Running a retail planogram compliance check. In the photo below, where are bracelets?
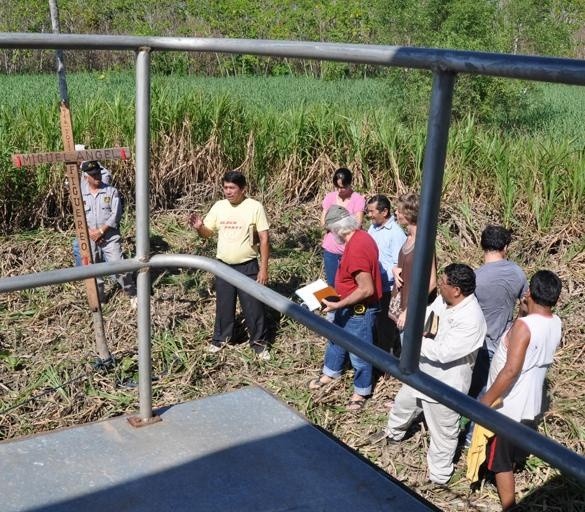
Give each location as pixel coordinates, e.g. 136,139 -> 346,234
261,266 -> 267,270
99,229 -> 105,236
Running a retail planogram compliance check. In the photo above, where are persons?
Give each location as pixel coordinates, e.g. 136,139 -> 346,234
188,171 -> 271,362
478,270 -> 563,512
367,195 -> 406,358
319,168 -> 366,321
66,144 -> 112,267
368,263 -> 488,493
463,226 -> 529,452
391,195 -> 438,349
79,160 -> 137,310
306,206 -> 382,413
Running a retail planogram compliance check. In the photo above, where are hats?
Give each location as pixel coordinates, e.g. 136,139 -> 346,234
323,205 -> 350,225
81,161 -> 103,172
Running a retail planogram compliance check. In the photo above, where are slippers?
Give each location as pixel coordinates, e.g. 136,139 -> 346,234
345,398 -> 367,410
309,379 -> 326,391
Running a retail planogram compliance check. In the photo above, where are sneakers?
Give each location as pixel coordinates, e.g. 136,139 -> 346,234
209,336 -> 231,353
369,431 -> 400,444
130,297 -> 155,316
258,351 -> 271,361
